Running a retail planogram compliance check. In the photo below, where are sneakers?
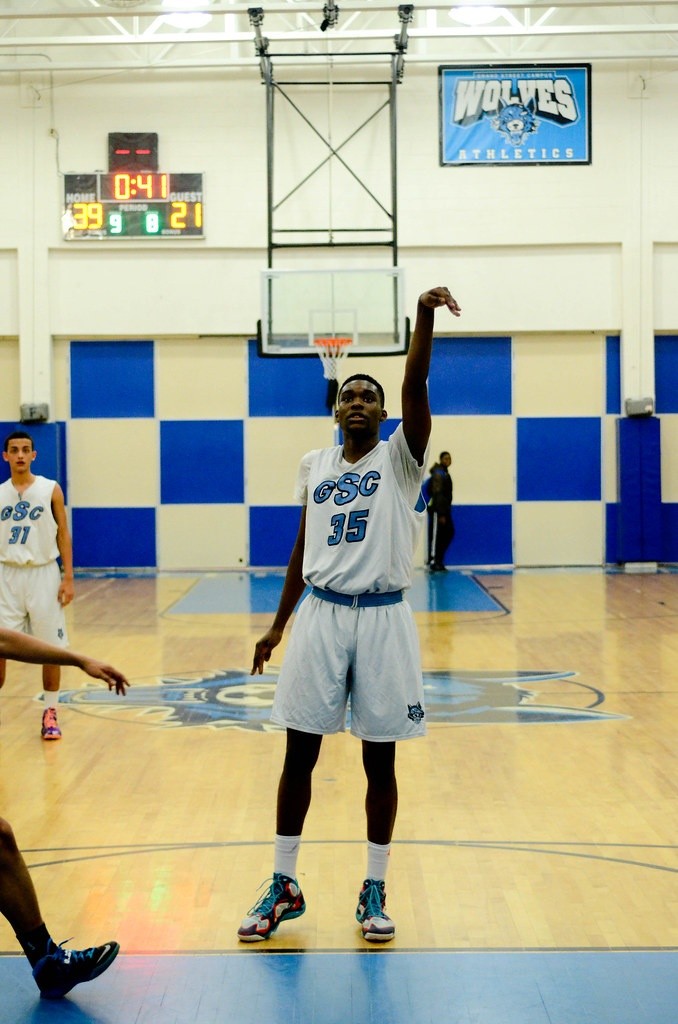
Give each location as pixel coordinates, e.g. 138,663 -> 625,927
41,707 -> 63,739
33,940 -> 119,998
356,877 -> 395,943
239,872 -> 304,942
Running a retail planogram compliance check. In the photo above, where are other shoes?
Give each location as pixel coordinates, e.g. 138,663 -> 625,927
430,564 -> 447,572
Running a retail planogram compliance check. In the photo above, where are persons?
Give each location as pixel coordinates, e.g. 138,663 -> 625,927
237,286 -> 461,944
0,431 -> 76,740
427,452 -> 455,573
0,625 -> 132,996
